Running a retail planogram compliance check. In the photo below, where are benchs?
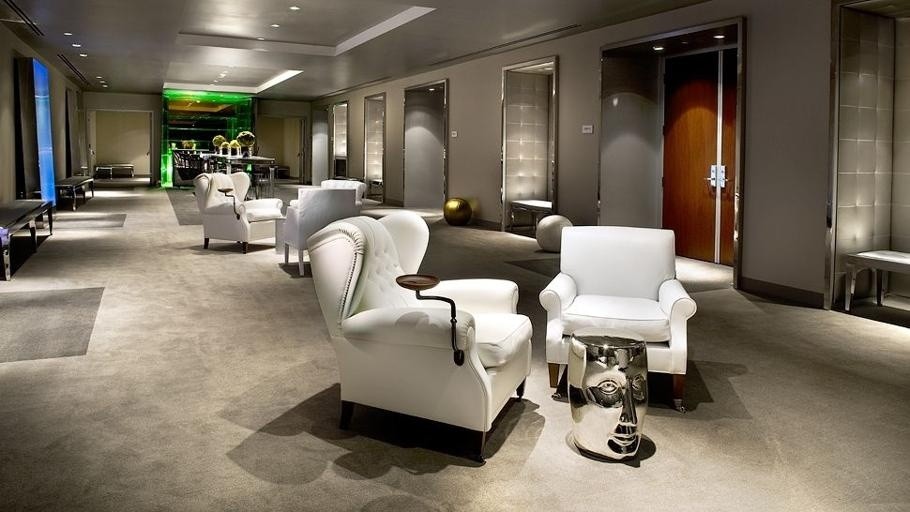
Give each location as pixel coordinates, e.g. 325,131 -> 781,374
844,250 -> 910,313
0,176 -> 95,281
94,163 -> 135,179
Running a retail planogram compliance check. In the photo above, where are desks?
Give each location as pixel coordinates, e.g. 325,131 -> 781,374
200,153 -> 276,174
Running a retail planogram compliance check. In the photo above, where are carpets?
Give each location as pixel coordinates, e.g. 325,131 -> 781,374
166,186 -> 204,226
0,286 -> 106,363
51,213 -> 127,228
